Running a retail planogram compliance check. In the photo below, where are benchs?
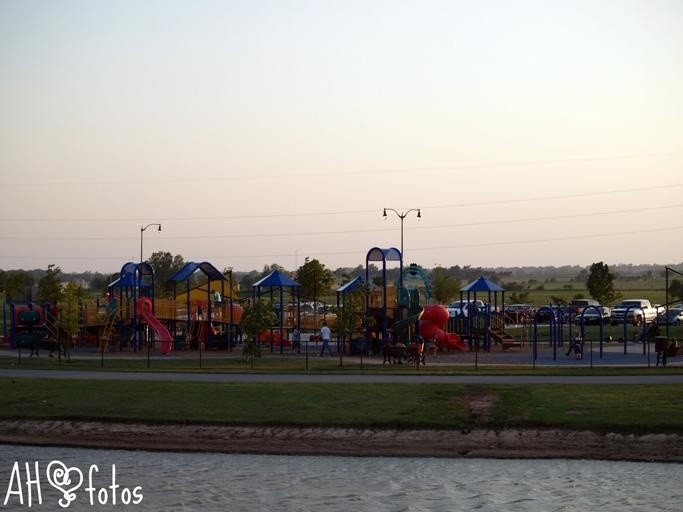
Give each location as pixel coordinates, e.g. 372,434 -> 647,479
662,346 -> 683,364
27,341 -> 61,358
383,347 -> 425,368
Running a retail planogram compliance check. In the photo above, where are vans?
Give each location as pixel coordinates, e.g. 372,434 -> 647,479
445,300 -> 485,319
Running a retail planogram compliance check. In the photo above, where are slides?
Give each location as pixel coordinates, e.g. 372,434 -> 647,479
142,314 -> 172,354
420,305 -> 467,353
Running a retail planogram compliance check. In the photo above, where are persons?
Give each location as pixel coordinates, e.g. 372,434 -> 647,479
29,332 -> 70,359
289,326 -> 302,352
314,320 -> 336,358
210,290 -> 222,309
381,332 -> 439,367
473,302 -> 583,362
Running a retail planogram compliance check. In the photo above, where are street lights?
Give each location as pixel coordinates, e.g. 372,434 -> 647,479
138,224 -> 162,352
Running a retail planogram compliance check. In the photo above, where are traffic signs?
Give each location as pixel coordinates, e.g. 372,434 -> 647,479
381,208 -> 421,364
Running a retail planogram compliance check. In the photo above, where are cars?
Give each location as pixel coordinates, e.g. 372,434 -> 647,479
171,302 -> 345,333
483,298 -> 683,326
